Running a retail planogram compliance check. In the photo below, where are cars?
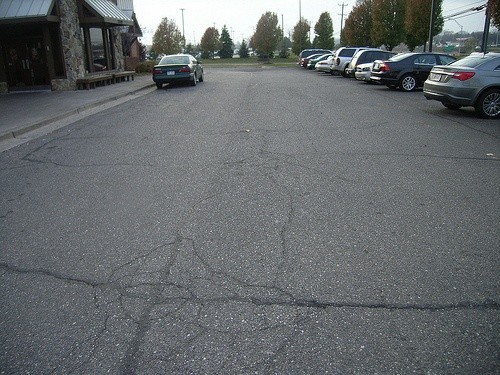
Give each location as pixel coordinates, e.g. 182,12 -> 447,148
153,55 -> 204,88
301,53 -> 334,73
345,50 -> 411,84
420,53 -> 500,119
370,52 -> 462,92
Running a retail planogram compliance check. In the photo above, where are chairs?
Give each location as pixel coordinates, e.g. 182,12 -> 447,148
421,56 -> 429,63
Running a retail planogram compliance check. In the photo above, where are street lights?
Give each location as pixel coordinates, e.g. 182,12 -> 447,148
181,9 -> 185,36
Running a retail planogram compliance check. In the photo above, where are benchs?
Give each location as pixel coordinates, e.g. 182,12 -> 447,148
106,71 -> 136,84
77,74 -> 113,90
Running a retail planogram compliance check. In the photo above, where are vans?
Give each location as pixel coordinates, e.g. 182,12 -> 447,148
299,49 -> 334,67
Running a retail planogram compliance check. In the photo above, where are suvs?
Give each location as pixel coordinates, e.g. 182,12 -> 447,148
330,46 -> 379,77
